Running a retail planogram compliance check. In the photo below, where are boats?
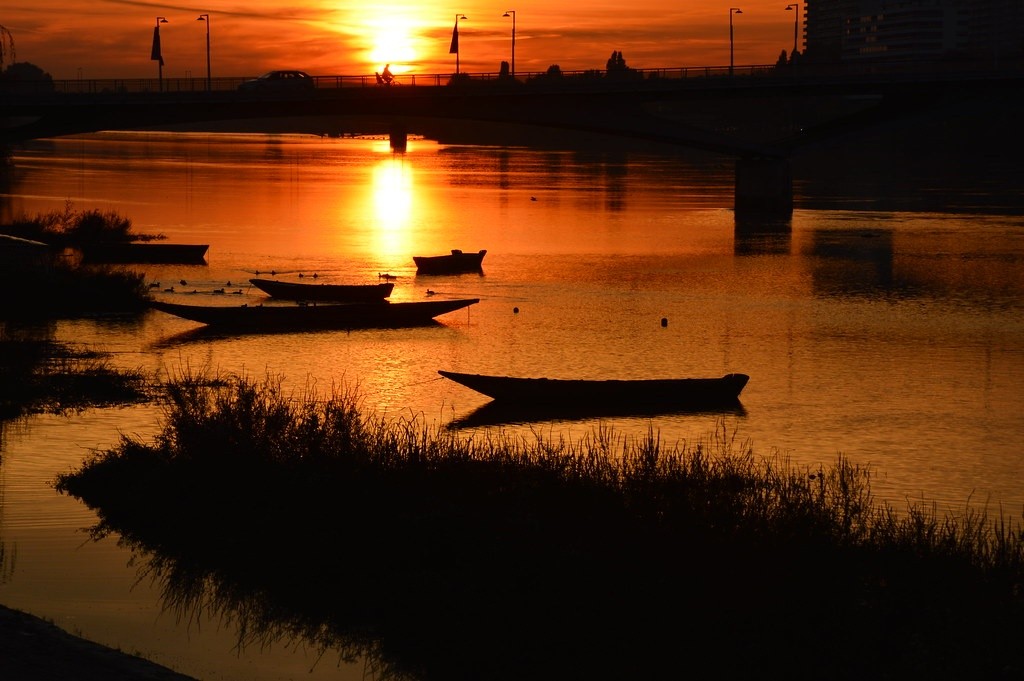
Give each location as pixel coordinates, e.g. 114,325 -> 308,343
437,369 -> 750,417
79,242 -> 210,262
246,279 -> 395,302
412,248 -> 488,277
147,297 -> 480,331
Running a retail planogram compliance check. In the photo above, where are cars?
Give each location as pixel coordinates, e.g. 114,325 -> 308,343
237,69 -> 315,93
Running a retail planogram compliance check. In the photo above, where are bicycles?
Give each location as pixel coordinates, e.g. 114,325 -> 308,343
375,77 -> 401,87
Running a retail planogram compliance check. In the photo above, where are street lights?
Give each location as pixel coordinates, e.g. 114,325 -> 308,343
455,13 -> 467,75
730,8 -> 744,69
197,14 -> 212,95
784,4 -> 799,57
157,15 -> 168,93
502,11 -> 516,75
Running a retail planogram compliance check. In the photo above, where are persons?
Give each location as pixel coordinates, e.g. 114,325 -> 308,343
375,72 -> 384,89
382,64 -> 394,86
